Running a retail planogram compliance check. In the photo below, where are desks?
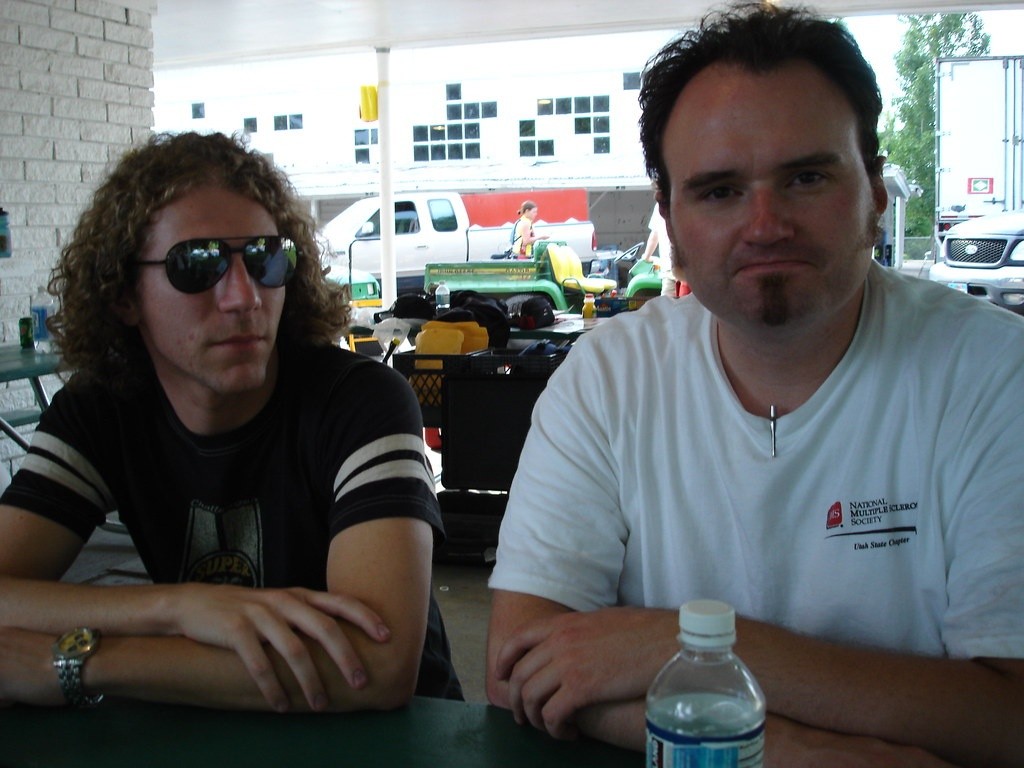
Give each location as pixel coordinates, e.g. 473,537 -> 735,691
0,691 -> 784,768
0,345 -> 74,415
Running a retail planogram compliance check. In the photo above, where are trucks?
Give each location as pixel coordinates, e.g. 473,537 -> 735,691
934,55 -> 1024,239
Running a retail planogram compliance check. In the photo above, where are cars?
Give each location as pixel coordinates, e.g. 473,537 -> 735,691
928,208 -> 1024,315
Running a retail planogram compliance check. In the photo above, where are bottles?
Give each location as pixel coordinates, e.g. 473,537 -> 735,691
582,294 -> 597,318
434,280 -> 450,309
645,599 -> 767,768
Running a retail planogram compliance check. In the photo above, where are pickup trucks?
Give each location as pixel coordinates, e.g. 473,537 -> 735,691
311,190 -> 598,293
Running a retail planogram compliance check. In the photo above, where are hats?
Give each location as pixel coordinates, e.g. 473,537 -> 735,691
374,293 -> 434,323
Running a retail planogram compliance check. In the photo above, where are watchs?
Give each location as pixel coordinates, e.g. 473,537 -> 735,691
52,626 -> 105,707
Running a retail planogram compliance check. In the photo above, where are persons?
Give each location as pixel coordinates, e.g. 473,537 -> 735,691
486,9 -> 1024,768
512,201 -> 550,259
641,201 -> 678,297
0,132 -> 466,714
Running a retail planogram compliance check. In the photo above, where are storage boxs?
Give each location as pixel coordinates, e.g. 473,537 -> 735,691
391,349 -> 573,426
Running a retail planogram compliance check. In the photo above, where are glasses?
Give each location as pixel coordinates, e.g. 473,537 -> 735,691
141,236 -> 299,294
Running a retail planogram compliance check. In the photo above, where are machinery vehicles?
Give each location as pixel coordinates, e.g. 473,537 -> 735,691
423,239 -> 662,316
318,263 -> 384,309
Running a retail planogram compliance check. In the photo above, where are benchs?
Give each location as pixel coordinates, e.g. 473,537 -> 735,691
0,409 -> 40,451
